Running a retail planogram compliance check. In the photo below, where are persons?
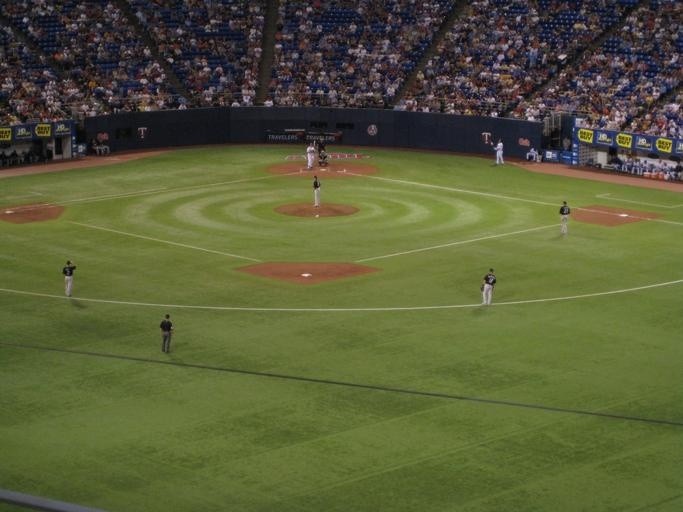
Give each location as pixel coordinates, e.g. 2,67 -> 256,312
559,200 -> 570,232
0,1 -> 683,183
159,314 -> 173,354
480,267 -> 496,306
312,175 -> 321,207
62,259 -> 76,297
